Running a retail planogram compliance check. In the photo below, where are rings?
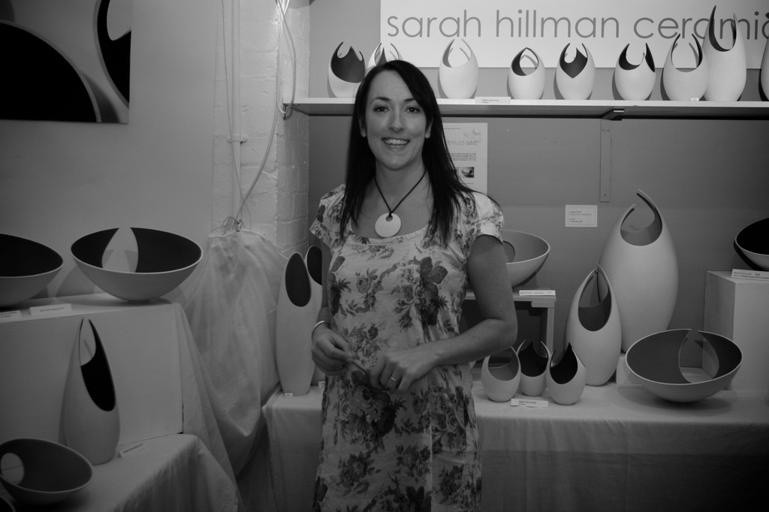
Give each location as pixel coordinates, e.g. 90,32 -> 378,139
391,376 -> 397,382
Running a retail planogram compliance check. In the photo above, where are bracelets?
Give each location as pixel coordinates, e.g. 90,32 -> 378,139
311,320 -> 330,345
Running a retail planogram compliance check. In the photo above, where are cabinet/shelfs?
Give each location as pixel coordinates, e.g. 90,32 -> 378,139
4,282 -> 198,511
250,96 -> 769,507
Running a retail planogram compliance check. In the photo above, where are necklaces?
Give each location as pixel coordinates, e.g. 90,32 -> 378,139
375,167 -> 428,238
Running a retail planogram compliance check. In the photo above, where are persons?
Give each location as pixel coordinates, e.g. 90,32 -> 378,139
311,59 -> 518,512
